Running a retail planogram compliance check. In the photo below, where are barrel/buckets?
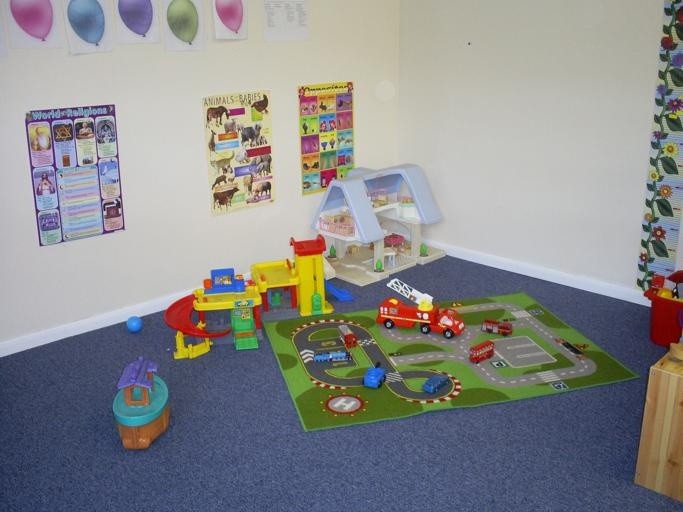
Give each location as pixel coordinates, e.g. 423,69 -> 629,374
645,288 -> 683,346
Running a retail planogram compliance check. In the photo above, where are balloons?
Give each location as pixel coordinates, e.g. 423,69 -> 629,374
10,0 -> 53,42
214,0 -> 243,35
165,1 -> 198,45
66,1 -> 104,48
117,0 -> 152,38
